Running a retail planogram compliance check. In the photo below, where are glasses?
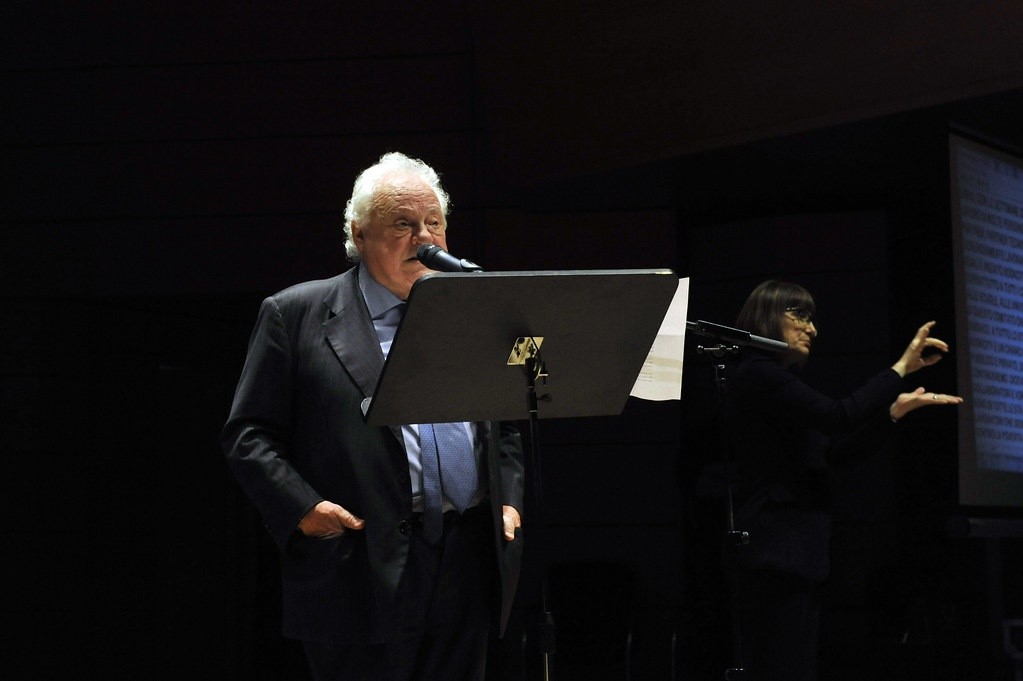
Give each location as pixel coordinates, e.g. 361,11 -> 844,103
786,305 -> 811,322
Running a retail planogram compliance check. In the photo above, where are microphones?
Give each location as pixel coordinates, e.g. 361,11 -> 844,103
417,244 -> 484,275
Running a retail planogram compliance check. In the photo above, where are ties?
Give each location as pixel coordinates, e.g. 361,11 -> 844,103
398,303 -> 480,546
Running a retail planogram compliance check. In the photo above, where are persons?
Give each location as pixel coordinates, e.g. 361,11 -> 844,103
700,279 -> 965,681
219,153 -> 527,681
